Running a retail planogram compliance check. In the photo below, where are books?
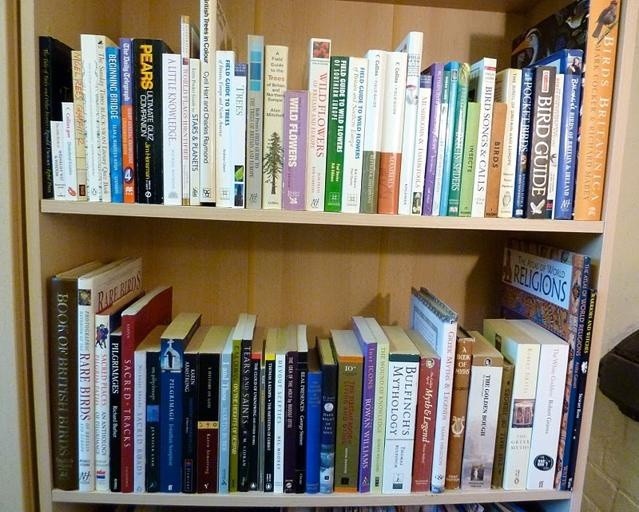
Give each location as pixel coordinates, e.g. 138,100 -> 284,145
44,242 -> 597,512
38,2 -> 621,221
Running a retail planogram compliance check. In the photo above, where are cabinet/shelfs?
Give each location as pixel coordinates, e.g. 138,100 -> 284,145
0,0 -> 639,512
